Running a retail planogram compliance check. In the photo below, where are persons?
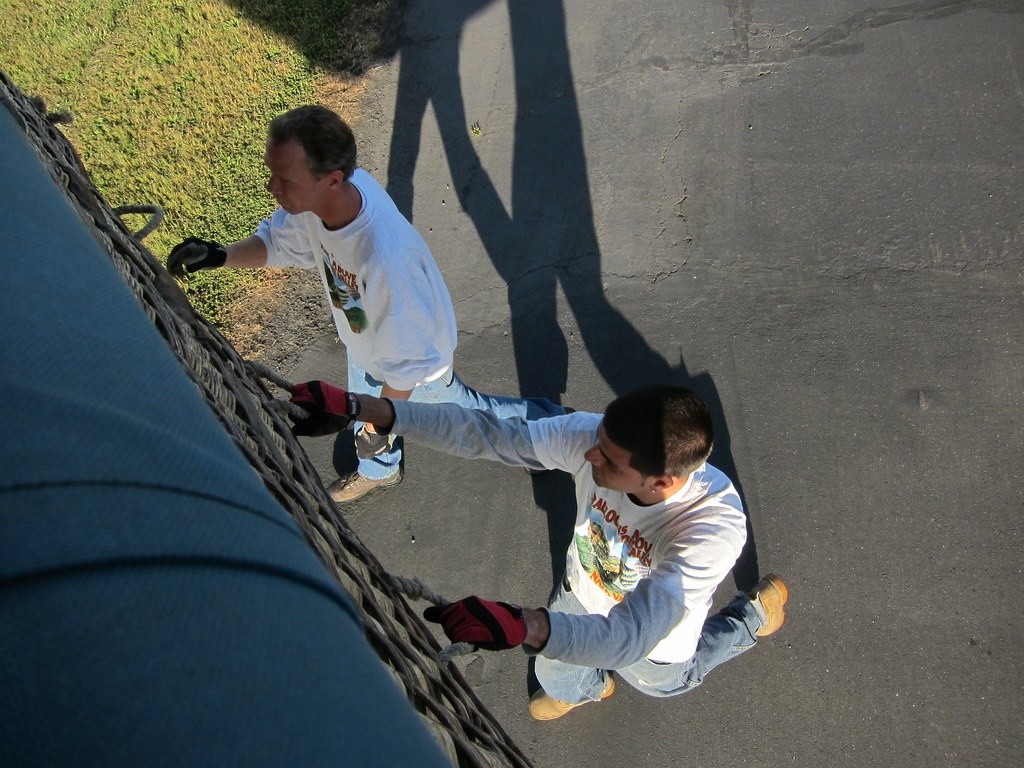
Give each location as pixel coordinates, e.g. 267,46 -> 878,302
288,384 -> 788,719
167,103 -> 457,504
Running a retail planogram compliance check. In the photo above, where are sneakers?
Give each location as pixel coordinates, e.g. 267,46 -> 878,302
325,467 -> 403,505
564,406 -> 576,414
527,670 -> 615,721
749,573 -> 788,636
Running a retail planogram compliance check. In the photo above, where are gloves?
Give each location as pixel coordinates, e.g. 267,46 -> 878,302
422,595 -> 528,653
286,380 -> 361,437
354,424 -> 393,459
166,235 -> 227,284
